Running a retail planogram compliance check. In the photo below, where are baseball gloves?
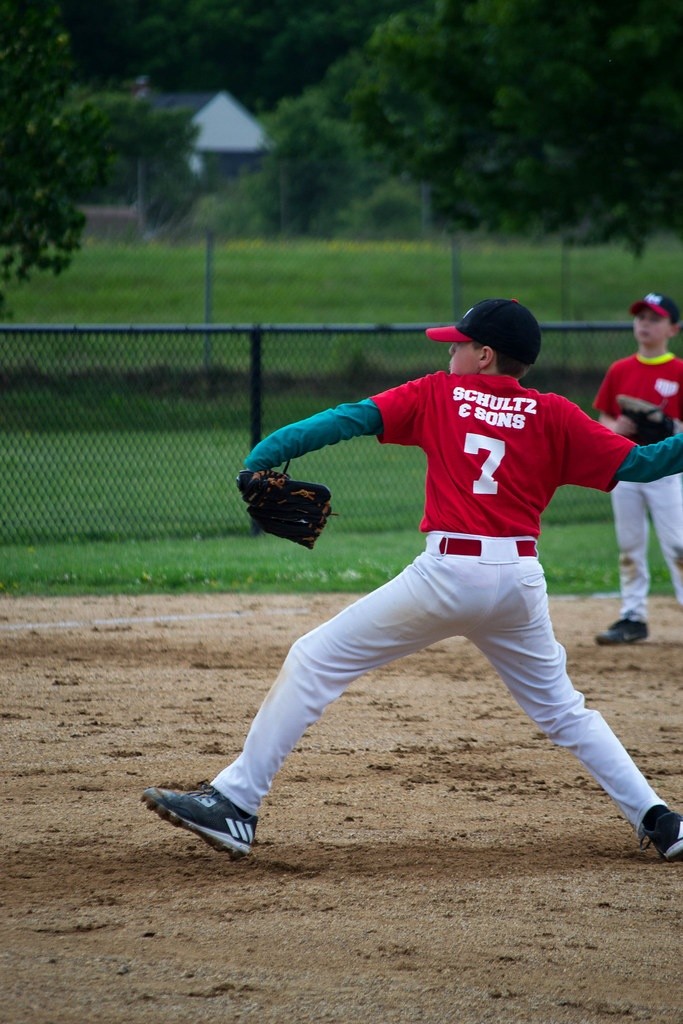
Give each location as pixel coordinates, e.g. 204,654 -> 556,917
236,458 -> 339,550
617,395 -> 674,444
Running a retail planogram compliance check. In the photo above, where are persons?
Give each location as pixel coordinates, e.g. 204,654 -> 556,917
592,293 -> 683,646
140,299 -> 683,858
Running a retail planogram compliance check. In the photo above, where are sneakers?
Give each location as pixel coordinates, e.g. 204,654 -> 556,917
141,784 -> 258,860
640,812 -> 683,860
596,617 -> 647,643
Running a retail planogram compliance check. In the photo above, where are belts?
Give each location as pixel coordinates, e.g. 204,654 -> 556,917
439,537 -> 536,557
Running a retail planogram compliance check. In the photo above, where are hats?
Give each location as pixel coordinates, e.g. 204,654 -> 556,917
425,298 -> 541,364
628,292 -> 679,324
